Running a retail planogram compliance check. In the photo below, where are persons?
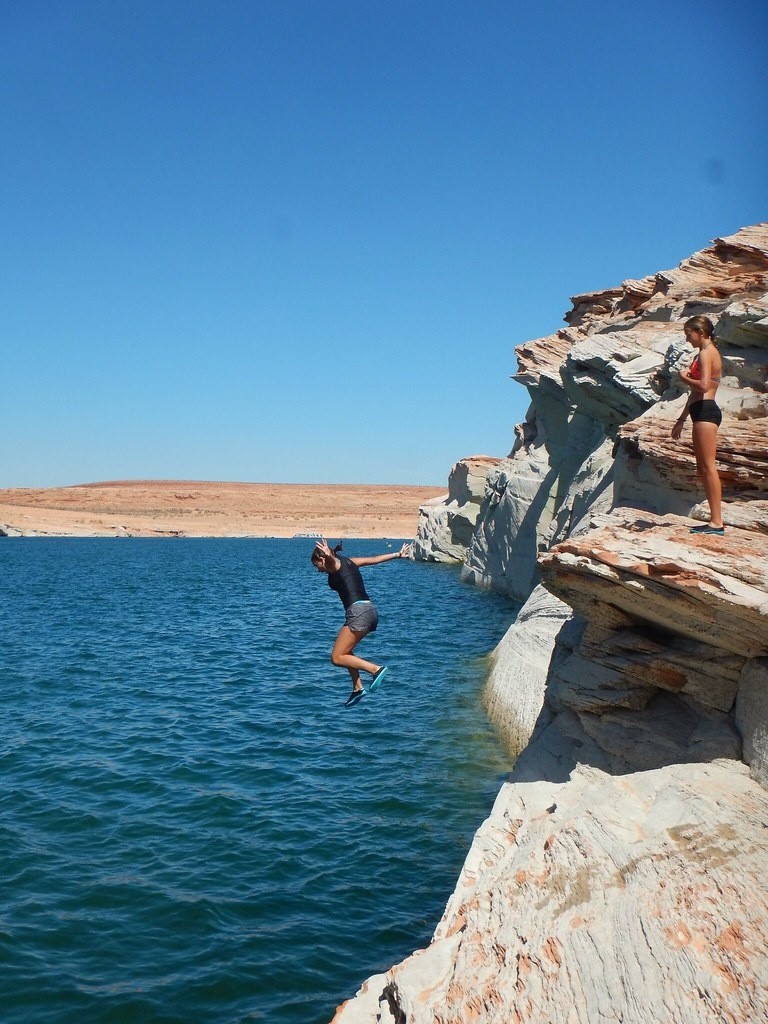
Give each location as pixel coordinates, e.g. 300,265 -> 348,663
311,539 -> 412,708
671,316 -> 732,537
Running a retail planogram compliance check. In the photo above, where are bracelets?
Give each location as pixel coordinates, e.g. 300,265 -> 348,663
676,418 -> 687,422
393,550 -> 402,559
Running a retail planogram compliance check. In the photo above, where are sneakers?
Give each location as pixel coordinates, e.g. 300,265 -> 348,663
344,687 -> 367,707
690,524 -> 724,536
369,666 -> 389,693
